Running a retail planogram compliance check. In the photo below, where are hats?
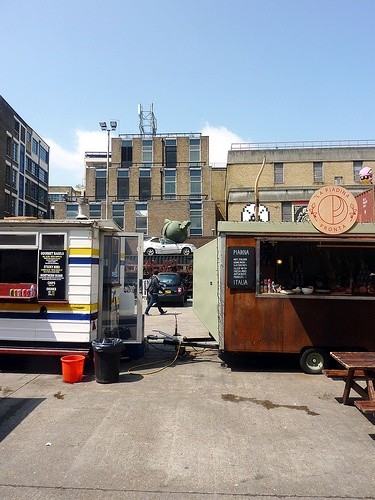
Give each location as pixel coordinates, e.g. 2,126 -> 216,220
152,269 -> 160,273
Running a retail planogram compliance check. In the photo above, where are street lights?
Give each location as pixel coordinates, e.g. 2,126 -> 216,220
99,121 -> 117,219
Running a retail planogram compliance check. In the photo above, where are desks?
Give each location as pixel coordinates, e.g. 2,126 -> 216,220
330,351 -> 375,417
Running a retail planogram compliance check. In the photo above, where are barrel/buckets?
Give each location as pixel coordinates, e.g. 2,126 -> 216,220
61,355 -> 85,383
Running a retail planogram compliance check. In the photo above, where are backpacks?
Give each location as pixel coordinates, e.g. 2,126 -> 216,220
146,277 -> 157,292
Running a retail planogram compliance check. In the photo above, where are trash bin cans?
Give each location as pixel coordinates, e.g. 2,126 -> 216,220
91,337 -> 123,384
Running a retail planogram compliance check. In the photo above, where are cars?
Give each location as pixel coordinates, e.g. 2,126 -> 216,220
147,272 -> 187,307
137,236 -> 198,256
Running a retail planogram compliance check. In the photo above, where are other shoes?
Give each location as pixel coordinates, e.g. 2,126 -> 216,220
160,310 -> 167,315
144,312 -> 150,316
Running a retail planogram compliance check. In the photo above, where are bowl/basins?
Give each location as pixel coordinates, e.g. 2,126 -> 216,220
301,287 -> 314,295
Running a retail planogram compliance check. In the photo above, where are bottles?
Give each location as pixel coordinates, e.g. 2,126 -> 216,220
30,284 -> 36,297
260,278 -> 273,293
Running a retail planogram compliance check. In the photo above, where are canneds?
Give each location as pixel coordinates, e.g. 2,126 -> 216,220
296,263 -> 304,286
260,279 -> 276,293
9,284 -> 37,297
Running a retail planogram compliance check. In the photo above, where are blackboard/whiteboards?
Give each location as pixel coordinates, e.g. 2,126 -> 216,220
228,246 -> 256,290
37,248 -> 68,302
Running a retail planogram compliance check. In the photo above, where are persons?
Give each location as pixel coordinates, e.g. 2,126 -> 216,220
144,269 -> 167,316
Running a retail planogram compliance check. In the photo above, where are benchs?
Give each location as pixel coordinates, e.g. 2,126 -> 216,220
353,400 -> 375,413
321,369 -> 375,382
